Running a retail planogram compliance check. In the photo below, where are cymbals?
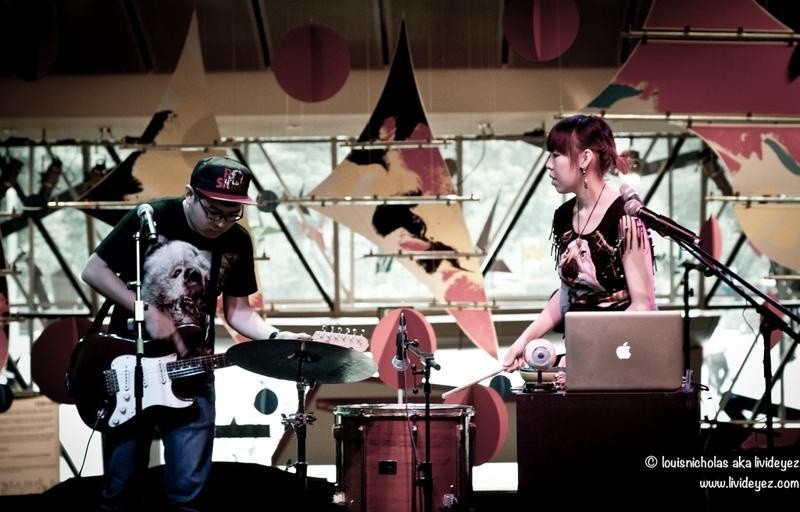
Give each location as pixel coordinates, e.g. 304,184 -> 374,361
227,340 -> 380,385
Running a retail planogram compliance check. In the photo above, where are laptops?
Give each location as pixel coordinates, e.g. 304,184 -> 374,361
564,312 -> 684,391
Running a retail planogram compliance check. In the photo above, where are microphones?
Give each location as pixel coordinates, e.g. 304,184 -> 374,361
619,184 -> 668,236
624,199 -> 704,246
392,313 -> 411,372
138,204 -> 158,245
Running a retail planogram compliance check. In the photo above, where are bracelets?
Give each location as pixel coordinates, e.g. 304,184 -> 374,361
269,330 -> 278,340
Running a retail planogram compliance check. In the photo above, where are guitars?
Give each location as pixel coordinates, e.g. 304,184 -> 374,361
68,324 -> 371,433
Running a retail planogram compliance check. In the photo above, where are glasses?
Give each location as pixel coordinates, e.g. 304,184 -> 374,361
196,193 -> 244,222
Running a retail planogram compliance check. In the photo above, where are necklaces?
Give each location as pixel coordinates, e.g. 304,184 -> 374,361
576,182 -> 606,245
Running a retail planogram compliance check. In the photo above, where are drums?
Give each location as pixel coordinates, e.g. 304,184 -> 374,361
333,402 -> 476,511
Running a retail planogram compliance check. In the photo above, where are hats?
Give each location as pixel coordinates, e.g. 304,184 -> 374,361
191,157 -> 256,205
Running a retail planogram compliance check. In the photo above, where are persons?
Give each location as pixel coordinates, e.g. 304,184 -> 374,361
81,157 -> 317,512
503,114 -> 658,373
